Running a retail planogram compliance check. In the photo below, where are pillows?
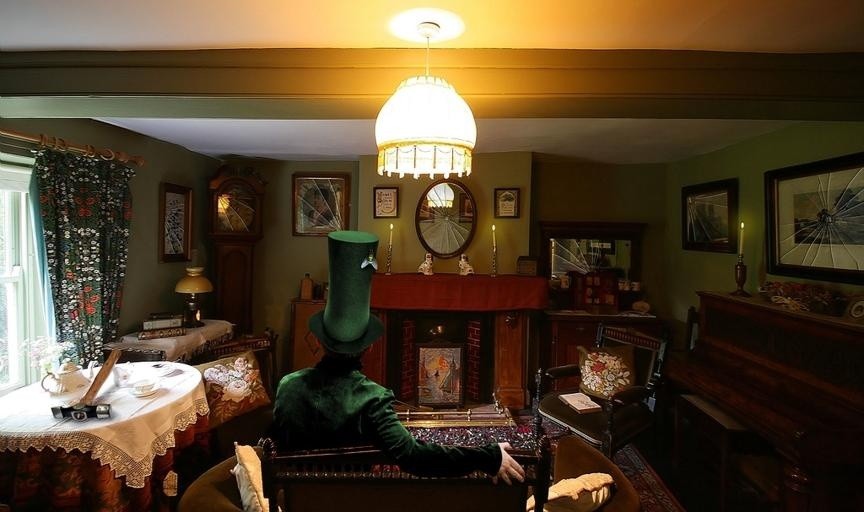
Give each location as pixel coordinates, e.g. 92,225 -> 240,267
192,349 -> 271,431
525,471 -> 616,512
229,440 -> 282,512
576,344 -> 636,401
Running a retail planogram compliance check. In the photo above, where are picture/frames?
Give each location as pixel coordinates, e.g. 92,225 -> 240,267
493,187 -> 521,219
418,198 -> 434,223
585,239 -> 615,255
373,186 -> 399,218
459,191 -> 473,224
681,176 -> 739,255
764,152 -> 864,286
159,181 -> 193,264
845,296 -> 864,325
291,174 -> 351,238
414,337 -> 466,408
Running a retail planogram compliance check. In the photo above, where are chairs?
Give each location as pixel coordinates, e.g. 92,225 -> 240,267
191,337 -> 277,458
532,318 -> 670,461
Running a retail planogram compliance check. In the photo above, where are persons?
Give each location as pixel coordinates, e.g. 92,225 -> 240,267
308,189 -> 335,226
273,336 -> 527,486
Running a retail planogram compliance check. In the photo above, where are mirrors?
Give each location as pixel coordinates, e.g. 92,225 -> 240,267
414,178 -> 476,259
539,221 -> 648,281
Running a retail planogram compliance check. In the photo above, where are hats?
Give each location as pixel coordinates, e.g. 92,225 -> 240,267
308,230 -> 386,355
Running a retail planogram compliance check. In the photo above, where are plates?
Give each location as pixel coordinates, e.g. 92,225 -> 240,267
130,386 -> 157,397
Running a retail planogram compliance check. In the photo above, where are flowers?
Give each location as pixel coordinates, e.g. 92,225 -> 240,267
19,335 -> 76,365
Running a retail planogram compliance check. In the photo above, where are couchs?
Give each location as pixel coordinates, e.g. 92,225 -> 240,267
174,434 -> 641,511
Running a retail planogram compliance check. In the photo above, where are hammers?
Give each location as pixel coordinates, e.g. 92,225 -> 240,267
51,349 -> 122,423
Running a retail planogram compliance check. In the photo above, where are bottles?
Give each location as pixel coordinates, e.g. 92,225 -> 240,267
300,273 -> 312,299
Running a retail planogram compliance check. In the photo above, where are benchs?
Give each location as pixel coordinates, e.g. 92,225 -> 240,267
260,439 -> 551,511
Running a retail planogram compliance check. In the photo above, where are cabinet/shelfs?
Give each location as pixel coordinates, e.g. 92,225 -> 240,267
539,302 -> 663,394
288,298 -> 327,374
699,289 -> 864,512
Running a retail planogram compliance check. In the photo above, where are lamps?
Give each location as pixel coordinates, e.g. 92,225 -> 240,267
175,267 -> 214,328
374,21 -> 477,180
426,183 -> 455,209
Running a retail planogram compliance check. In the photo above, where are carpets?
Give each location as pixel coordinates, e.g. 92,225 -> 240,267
405,414 -> 688,512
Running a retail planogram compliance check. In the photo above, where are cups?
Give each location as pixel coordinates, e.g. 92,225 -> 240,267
135,381 -> 154,391
113,366 -> 130,386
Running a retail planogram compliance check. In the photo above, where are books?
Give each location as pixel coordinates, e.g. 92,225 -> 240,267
142,315 -> 183,329
558,393 -> 604,415
137,327 -> 186,340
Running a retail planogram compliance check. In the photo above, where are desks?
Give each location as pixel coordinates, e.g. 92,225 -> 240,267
102,318 -> 235,365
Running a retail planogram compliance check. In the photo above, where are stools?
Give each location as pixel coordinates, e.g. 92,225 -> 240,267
680,393 -> 753,512
731,448 -> 786,511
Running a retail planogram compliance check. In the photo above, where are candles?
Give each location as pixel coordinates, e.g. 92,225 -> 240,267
492,225 -> 496,248
389,224 -> 393,246
740,221 -> 745,253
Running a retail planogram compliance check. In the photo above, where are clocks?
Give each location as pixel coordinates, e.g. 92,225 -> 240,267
207,164 -> 265,242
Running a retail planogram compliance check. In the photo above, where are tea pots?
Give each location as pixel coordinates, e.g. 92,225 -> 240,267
41,358 -> 97,401
